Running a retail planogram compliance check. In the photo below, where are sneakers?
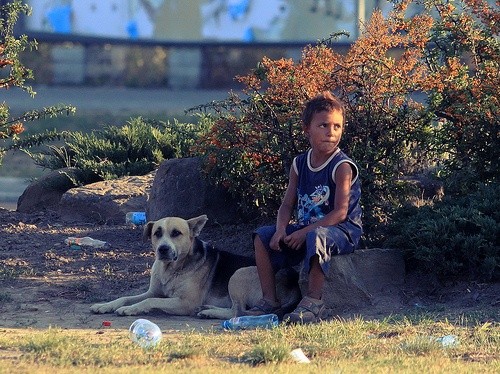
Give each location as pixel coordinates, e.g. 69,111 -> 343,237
238,299 -> 283,316
283,296 -> 328,324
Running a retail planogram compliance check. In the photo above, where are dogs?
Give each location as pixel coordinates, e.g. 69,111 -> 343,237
90,215 -> 303,319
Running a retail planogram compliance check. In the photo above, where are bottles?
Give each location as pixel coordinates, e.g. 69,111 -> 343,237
427,335 -> 457,347
128,319 -> 162,348
126,212 -> 146,224
221,314 -> 278,330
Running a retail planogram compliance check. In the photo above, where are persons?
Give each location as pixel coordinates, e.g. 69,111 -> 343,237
237,94 -> 362,326
